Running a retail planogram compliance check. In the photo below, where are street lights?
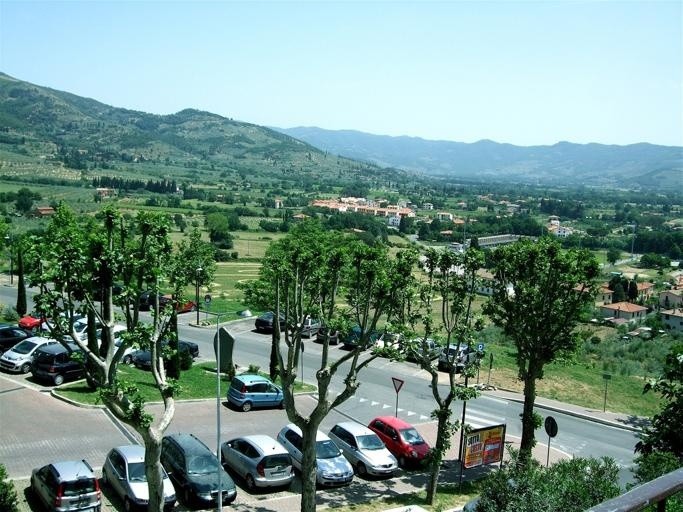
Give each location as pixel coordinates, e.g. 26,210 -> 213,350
195,267 -> 203,325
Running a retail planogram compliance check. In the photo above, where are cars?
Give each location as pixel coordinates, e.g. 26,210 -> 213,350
30,459 -> 102,512
255,311 -> 291,333
102,432 -> 237,512
410,337 -> 445,362
293,314 -> 378,351
226,374 -> 286,411
0,304 -> 199,386
133,289 -> 198,314
68,276 -> 123,302
438,344 -> 478,373
374,328 -> 405,355
216,415 -> 435,492
